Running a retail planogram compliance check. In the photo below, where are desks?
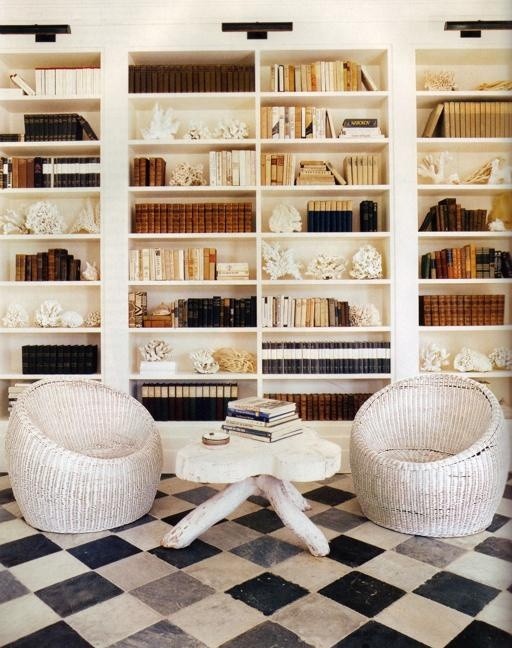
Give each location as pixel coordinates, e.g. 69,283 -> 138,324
161,427 -> 341,559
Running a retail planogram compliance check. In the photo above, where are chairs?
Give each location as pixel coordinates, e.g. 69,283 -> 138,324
4,377 -> 163,535
347,373 -> 510,538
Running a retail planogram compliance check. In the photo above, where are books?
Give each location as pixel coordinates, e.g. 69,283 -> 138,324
128,64 -> 254,93
2,155 -> 101,188
130,247 -> 250,280
2,66 -> 100,141
261,295 -> 391,374
422,101 -> 512,138
220,395 -> 305,444
259,62 -> 387,138
261,152 -> 379,232
140,383 -> 238,421
129,289 -> 258,327
8,345 -> 97,413
420,244 -> 512,279
135,203 -> 252,233
264,392 -> 376,422
418,198 -> 488,232
134,149 -> 256,186
419,296 -> 506,326
16,248 -> 80,281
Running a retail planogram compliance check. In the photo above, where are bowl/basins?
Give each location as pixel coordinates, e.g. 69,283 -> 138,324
202,432 -> 230,449
